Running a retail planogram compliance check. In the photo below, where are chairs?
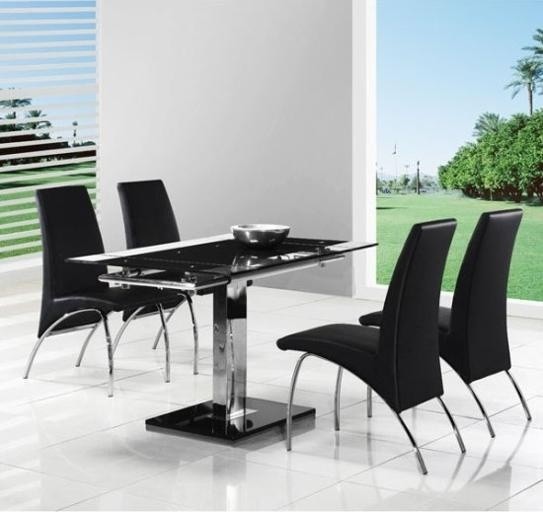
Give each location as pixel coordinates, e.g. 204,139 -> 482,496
357,207 -> 532,438
22,184 -> 173,398
275,217 -> 465,476
110,178 -> 253,376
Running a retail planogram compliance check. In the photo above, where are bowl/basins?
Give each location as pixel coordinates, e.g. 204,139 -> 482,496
232,223 -> 290,248
